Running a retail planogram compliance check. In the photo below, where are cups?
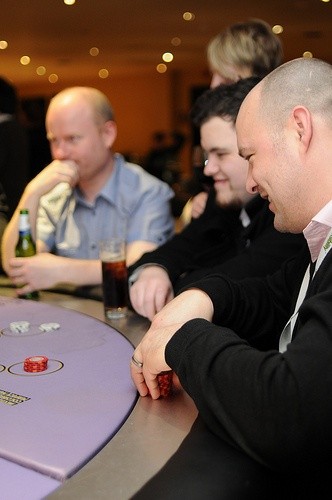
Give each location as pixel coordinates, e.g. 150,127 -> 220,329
100,238 -> 128,318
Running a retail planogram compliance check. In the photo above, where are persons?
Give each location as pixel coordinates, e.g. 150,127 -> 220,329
131,58 -> 332,500
208,20 -> 283,85
128,73 -> 309,354
0,75 -> 24,213
1,85 -> 178,297
141,130 -> 188,179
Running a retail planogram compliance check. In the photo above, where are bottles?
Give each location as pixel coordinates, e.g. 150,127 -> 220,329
14,210 -> 40,302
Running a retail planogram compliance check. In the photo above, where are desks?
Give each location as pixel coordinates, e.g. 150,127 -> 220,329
0,285 -> 199,500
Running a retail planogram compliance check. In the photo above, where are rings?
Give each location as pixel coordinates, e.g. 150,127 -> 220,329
132,355 -> 144,368
